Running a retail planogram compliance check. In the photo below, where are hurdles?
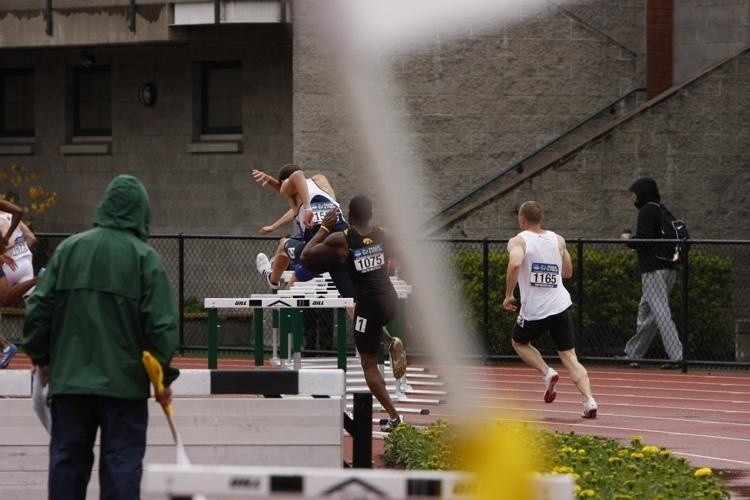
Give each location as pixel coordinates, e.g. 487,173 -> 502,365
144,463 -> 573,499
205,267 -> 447,425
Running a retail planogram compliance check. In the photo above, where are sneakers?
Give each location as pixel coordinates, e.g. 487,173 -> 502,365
389,336 -> 407,378
0,343 -> 17,368
580,397 -> 597,418
544,368 -> 559,403
616,355 -> 639,368
660,361 -> 681,370
256,253 -> 280,289
381,416 -> 403,432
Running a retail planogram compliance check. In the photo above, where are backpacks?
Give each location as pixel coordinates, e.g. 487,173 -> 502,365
656,207 -> 691,264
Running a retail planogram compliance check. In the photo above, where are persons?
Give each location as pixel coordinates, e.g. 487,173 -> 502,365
256,164 -> 407,377
22,173 -> 181,499
252,169 -> 295,234
0,198 -> 49,370
501,199 -> 598,419
614,176 -> 684,368
299,194 -> 407,431
0,210 -> 45,311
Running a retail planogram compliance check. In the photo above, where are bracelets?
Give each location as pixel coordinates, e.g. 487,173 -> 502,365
268,175 -> 272,184
304,207 -> 312,211
320,226 -> 330,233
